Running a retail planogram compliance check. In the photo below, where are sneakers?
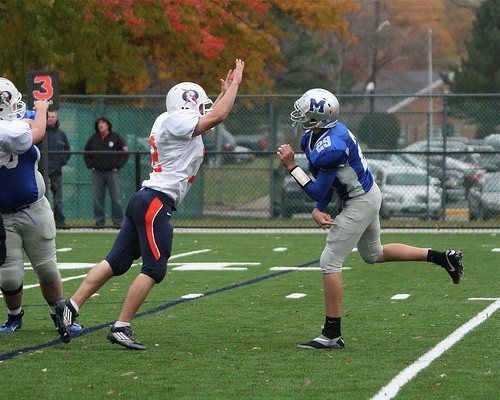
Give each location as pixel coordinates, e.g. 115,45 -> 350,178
0,310 -> 24,333
70,322 -> 83,332
55,299 -> 79,343
107,325 -> 146,350
442,250 -> 463,284
296,332 -> 344,349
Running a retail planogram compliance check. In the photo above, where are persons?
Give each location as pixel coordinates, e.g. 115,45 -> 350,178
83,117 -> 130,229
53,59 -> 244,350
276,88 -> 463,350
37,106 -> 72,229
0,76 -> 82,333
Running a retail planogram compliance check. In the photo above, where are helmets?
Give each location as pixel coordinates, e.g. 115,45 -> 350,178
0,77 -> 26,120
166,82 -> 213,116
291,89 -> 339,128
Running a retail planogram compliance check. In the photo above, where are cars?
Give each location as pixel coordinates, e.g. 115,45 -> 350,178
124,134 -> 152,165
202,124 -> 266,169
273,154 -> 341,219
366,133 -> 500,204
468,171 -> 500,222
374,166 -> 441,221
